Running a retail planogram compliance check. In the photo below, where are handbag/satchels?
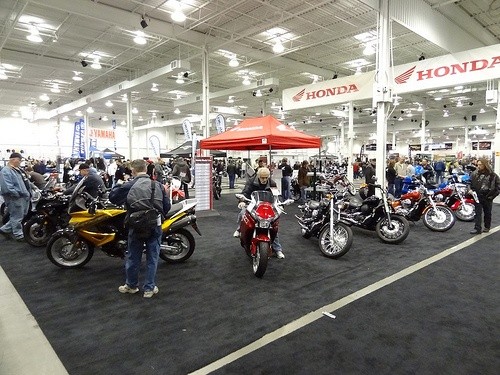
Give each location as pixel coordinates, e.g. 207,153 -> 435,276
128,208 -> 159,239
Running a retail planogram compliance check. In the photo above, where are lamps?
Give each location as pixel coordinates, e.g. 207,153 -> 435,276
0,0 -> 500,126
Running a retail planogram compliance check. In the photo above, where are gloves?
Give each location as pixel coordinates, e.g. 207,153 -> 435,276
237,202 -> 246,209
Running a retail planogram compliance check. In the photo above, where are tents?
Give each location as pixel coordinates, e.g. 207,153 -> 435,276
102,148 -> 125,160
160,141 -> 227,161
199,115 -> 322,203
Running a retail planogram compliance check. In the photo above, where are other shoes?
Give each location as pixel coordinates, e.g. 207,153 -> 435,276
483,227 -> 489,232
16,236 -> 26,242
470,229 -> 481,234
0,228 -> 13,241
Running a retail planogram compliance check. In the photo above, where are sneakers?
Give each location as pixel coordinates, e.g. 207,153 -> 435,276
275,251 -> 285,259
144,286 -> 159,298
233,230 -> 240,238
118,283 -> 139,294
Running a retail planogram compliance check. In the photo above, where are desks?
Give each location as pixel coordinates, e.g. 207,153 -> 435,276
303,186 -> 326,201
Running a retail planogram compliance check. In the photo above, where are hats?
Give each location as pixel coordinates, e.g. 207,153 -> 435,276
75,164 -> 90,171
10,152 -> 24,159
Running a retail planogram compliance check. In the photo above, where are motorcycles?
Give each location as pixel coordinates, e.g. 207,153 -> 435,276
212,168 -> 226,200
234,191 -> 295,278
0,156 -> 202,270
289,164 -> 476,259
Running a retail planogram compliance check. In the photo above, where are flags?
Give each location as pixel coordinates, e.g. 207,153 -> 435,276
150,136 -> 160,155
183,119 -> 192,140
216,114 -> 226,133
72,119 -> 86,158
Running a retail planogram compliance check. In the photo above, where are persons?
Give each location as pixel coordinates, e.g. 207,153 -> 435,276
386,157 -> 476,198
353,158 -> 377,196
146,157 -> 192,199
0,152 -> 132,242
272,157 -> 348,200
109,159 -> 171,297
470,159 -> 500,234
233,168 -> 284,257
213,159 -> 263,189
